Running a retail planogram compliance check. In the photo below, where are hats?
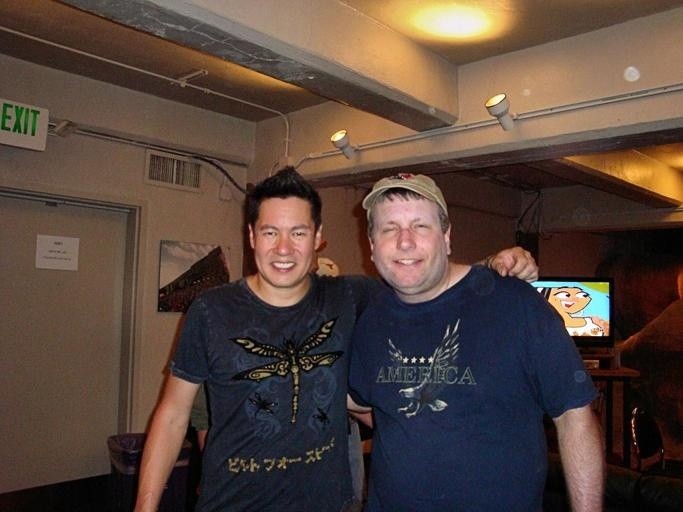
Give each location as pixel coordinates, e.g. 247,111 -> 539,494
362,172 -> 449,220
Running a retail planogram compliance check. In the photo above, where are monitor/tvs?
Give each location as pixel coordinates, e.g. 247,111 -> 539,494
525,277 -> 616,347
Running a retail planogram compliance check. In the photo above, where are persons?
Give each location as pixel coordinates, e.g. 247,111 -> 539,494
187,237 -> 373,510
133,163 -> 539,511
314,171 -> 608,511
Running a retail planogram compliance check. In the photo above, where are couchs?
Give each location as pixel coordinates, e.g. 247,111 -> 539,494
538,453 -> 682,510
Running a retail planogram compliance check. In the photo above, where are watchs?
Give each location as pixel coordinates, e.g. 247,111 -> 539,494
485,250 -> 497,268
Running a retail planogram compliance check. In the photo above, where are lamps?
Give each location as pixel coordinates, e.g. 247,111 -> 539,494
484,93 -> 515,131
330,128 -> 356,160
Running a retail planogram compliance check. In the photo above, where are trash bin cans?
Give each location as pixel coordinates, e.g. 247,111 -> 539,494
108,433 -> 192,512
